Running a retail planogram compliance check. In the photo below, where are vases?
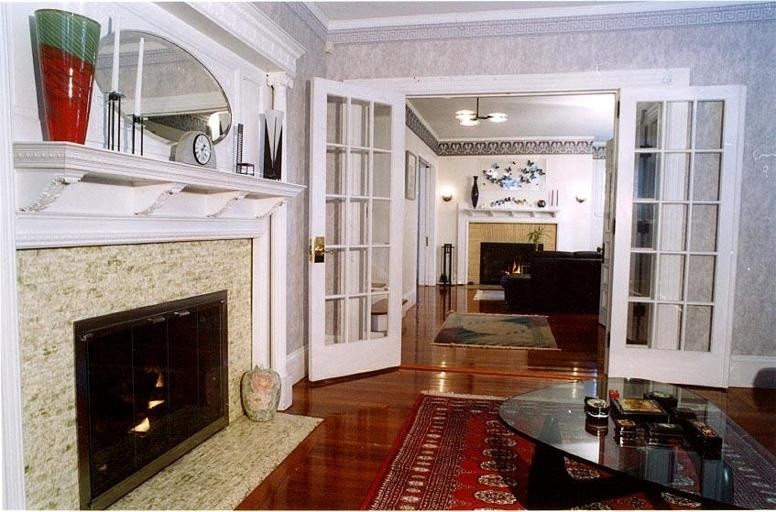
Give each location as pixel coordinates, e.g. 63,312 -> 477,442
471,175 -> 478,208
34,7 -> 105,142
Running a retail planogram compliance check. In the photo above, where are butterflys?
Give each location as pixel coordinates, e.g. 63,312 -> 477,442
481,159 -> 546,190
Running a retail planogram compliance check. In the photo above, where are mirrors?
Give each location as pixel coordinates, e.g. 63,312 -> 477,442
94,28 -> 232,149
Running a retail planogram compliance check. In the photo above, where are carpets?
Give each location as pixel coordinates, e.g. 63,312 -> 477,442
427,309 -> 563,351
473,289 -> 506,300
360,386 -> 775,508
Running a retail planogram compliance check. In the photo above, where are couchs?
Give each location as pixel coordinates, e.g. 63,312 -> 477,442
502,250 -> 603,314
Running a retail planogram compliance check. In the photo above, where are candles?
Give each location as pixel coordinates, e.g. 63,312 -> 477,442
134,37 -> 144,117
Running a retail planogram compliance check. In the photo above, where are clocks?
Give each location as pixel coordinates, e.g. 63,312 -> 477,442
178,129 -> 219,166
111,11 -> 121,92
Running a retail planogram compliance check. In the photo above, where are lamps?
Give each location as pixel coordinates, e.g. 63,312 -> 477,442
454,98 -> 509,127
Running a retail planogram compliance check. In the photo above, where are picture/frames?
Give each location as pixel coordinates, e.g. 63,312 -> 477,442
405,150 -> 418,200
